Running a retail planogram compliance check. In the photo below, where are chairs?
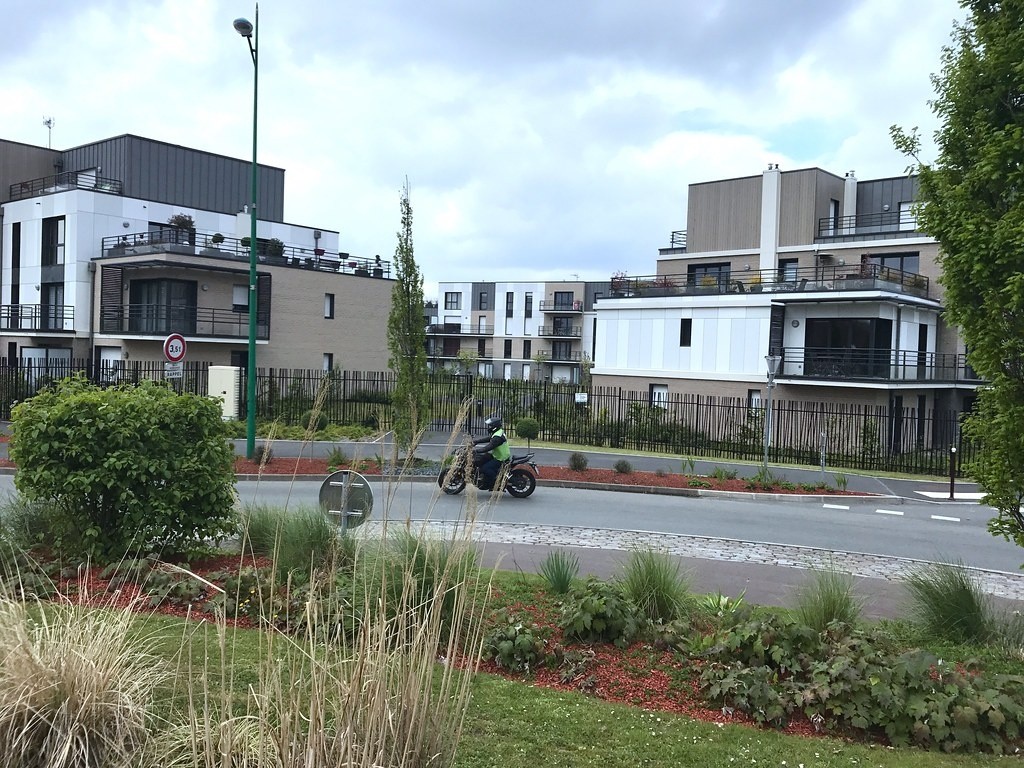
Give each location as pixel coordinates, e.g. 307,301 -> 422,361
736,281 -> 749,292
283,256 -> 300,268
793,279 -> 808,291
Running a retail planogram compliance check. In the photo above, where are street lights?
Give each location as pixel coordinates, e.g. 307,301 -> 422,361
232,16 -> 263,459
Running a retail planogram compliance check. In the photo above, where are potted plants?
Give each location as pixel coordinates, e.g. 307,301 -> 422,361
609,270 -> 719,297
373,255 -> 383,278
878,270 -> 927,298
108,212 -> 285,266
355,265 -> 369,277
750,275 -> 763,292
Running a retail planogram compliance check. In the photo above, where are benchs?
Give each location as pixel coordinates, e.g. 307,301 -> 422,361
316,258 -> 341,272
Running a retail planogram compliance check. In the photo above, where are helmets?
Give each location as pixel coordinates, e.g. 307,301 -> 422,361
485,418 -> 502,433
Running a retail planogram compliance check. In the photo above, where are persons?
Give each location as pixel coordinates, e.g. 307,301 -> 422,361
472,417 -> 510,490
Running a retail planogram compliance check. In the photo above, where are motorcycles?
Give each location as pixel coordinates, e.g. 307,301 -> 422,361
438,432 -> 539,498
